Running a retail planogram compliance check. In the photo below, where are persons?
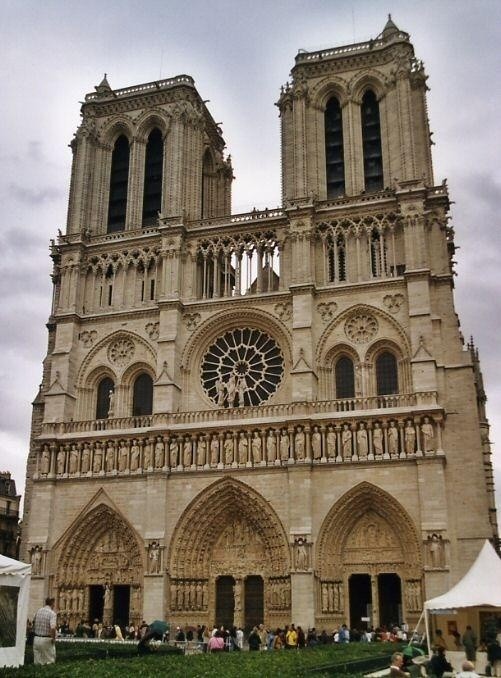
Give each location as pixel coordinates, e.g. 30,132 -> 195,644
432,624 -> 461,650
390,651 -> 409,677
463,624 -> 477,661
455,661 -> 480,678
430,645 -> 453,678
32,596 -> 57,665
487,639 -> 501,677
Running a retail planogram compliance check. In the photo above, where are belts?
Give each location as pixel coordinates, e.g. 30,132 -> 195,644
35,635 -> 52,637
